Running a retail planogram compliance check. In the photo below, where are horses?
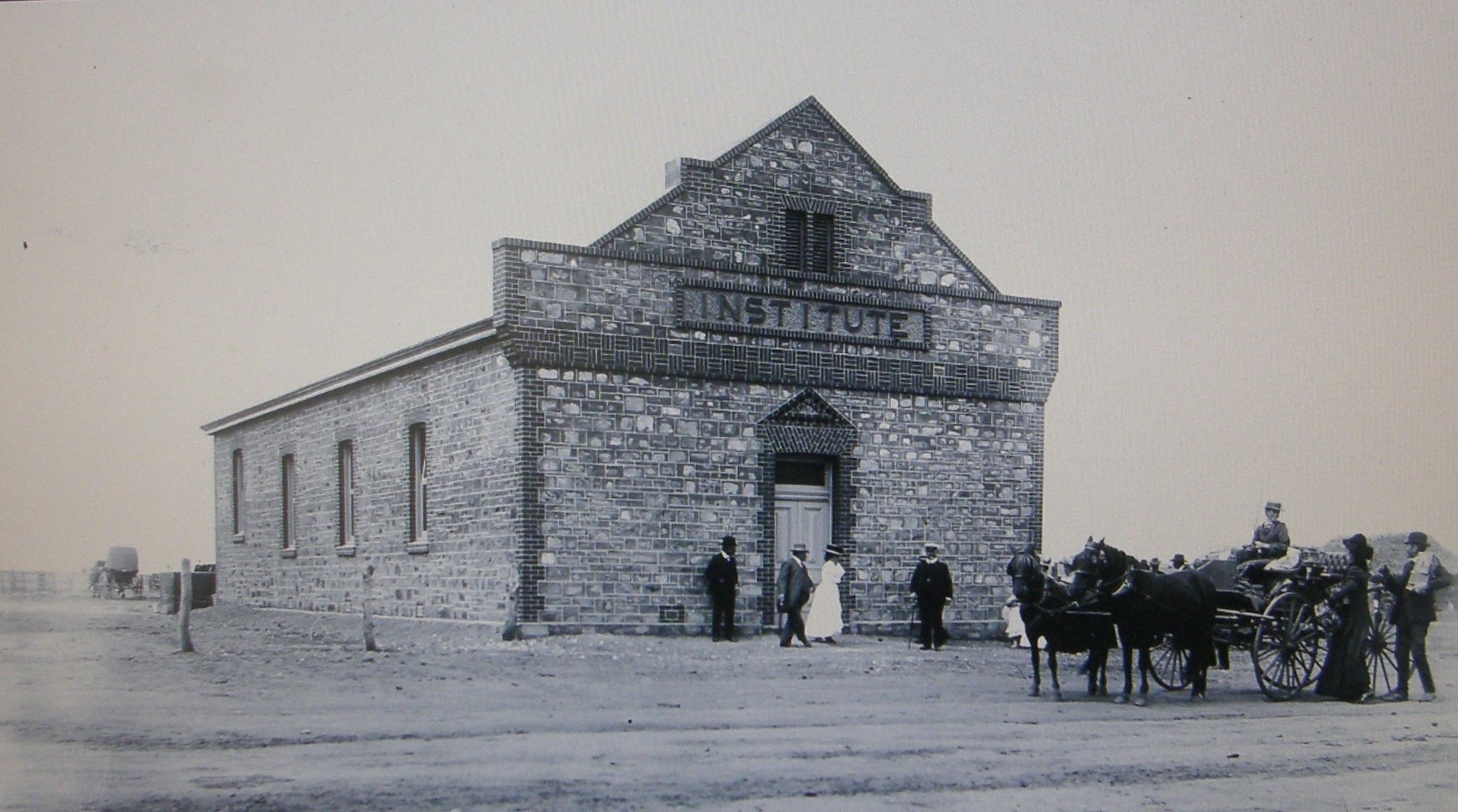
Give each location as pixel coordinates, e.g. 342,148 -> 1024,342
89,560 -> 107,599
1007,542 -> 1128,701
1069,536 -> 1217,706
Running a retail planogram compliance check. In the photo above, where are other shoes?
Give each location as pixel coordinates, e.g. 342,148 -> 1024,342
814,637 -> 836,643
725,635 -> 737,642
934,644 -> 946,650
1214,664 -> 1230,670
1382,692 -> 1409,701
1357,691 -> 1375,703
780,641 -> 792,646
920,645 -> 931,650
802,640 -> 812,647
711,633 -> 719,642
1418,692 -> 1437,701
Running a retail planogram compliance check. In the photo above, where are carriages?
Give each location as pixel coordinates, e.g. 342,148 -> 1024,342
1002,535 -> 1416,703
86,546 -> 145,600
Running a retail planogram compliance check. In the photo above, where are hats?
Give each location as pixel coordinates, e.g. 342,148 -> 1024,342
1150,557 -> 1162,565
720,536 -> 737,546
1402,532 -> 1430,552
790,544 -> 808,552
823,543 -> 844,555
1265,502 -> 1285,512
1169,554 -> 1188,562
1342,533 -> 1373,560
925,544 -> 938,552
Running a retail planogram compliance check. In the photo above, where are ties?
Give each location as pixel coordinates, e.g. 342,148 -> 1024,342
801,562 -> 805,568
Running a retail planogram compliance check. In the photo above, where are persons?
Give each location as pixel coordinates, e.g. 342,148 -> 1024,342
804,544 -> 846,644
705,536 -> 739,643
1315,533 -> 1375,703
908,543 -> 954,652
777,543 -> 815,648
1003,552 -> 1230,670
1379,532 -> 1453,702
1233,501 -> 1291,584
